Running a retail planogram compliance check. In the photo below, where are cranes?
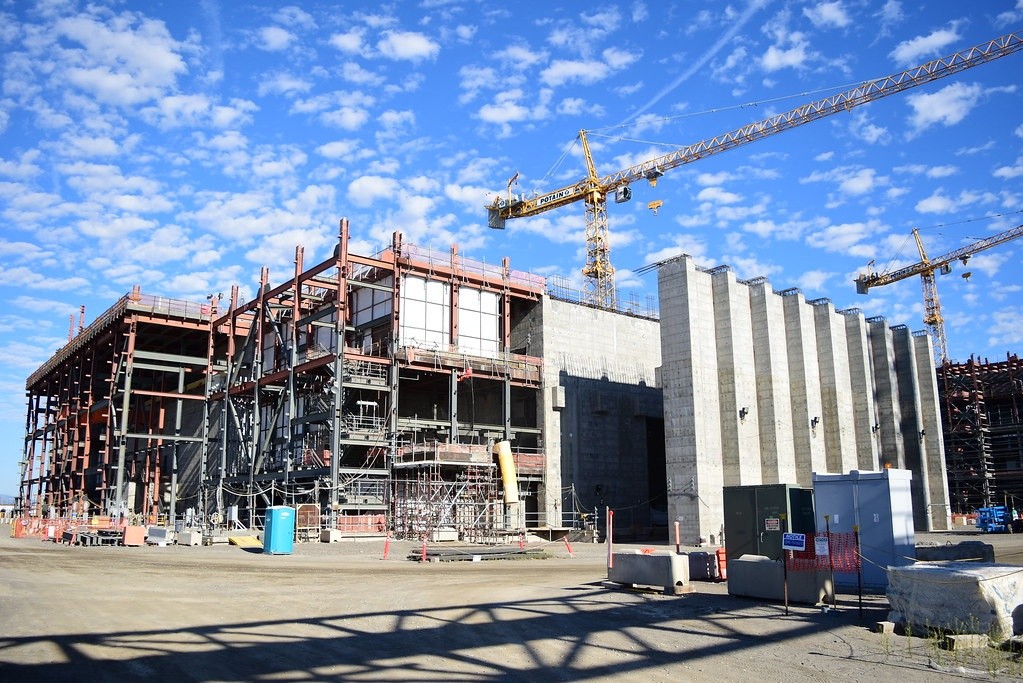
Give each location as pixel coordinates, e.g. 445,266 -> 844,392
853,209 -> 1023,367
487,27 -> 1023,307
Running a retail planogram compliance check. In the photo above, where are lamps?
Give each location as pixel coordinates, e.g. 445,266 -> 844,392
919,430 -> 927,439
812,417 -> 819,428
873,424 -> 880,433
740,407 -> 749,417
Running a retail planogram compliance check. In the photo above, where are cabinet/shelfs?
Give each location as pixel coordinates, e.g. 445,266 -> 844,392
811,467 -> 916,592
724,484 -> 835,603
